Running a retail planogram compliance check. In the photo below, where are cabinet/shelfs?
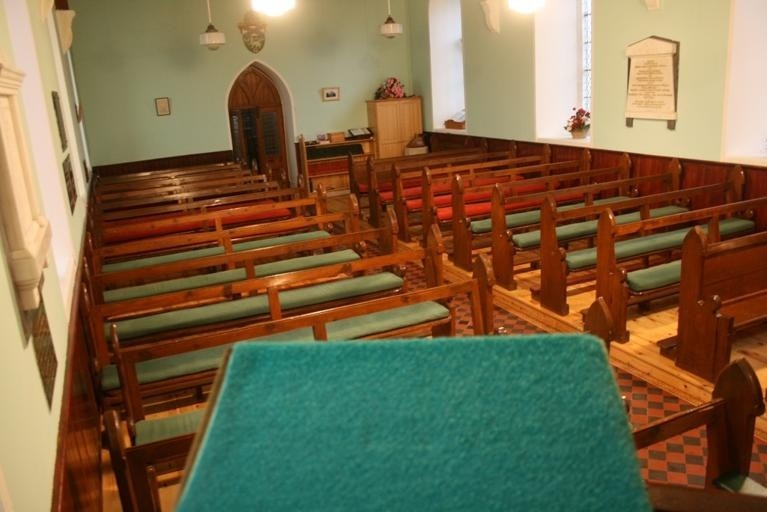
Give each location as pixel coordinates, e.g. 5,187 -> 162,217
293,132 -> 377,200
365,95 -> 423,160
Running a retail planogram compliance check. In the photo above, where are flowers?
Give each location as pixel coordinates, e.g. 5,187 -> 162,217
563,106 -> 590,132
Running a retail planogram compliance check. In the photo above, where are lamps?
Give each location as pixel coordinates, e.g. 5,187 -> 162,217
379,0 -> 404,40
198,0 -> 228,51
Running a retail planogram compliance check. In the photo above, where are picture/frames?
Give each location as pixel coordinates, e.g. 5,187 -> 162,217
320,86 -> 341,102
154,96 -> 172,117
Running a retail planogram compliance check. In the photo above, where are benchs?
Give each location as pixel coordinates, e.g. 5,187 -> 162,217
77,137 -> 764,511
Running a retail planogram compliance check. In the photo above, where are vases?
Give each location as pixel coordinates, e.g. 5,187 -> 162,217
570,129 -> 588,139
